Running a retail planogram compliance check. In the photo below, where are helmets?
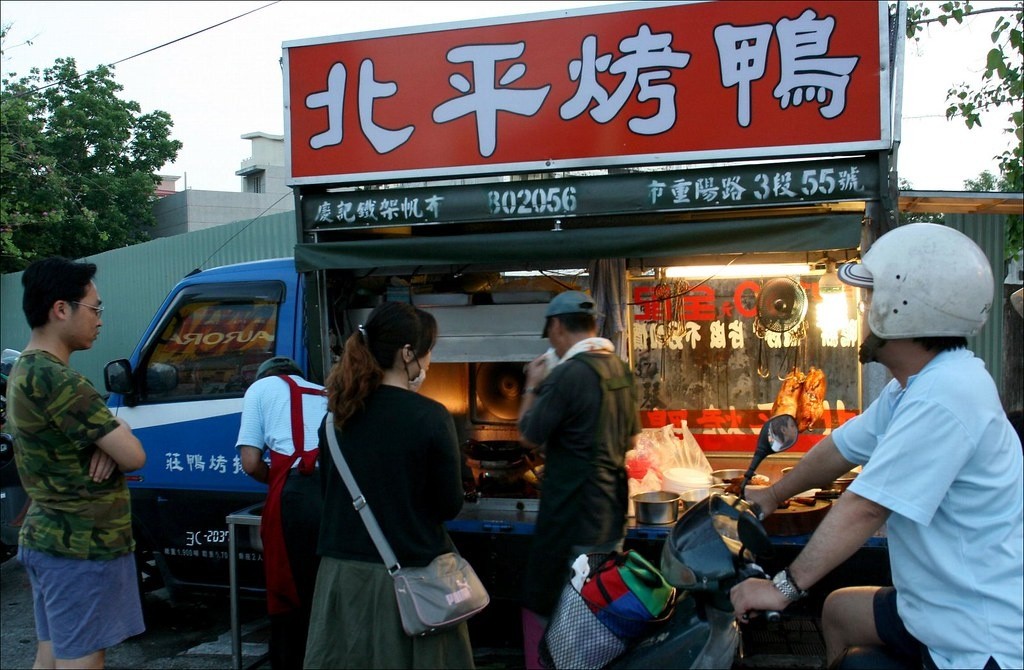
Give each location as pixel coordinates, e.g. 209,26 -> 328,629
840,221 -> 995,341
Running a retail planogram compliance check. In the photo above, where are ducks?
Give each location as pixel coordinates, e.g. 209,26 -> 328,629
770,366 -> 827,433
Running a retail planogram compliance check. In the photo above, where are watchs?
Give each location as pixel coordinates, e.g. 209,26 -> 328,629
773,570 -> 799,600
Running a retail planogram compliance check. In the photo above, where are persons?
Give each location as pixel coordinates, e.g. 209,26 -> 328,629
304,300 -> 475,670
234,357 -> 329,670
6,257 -> 146,670
518,291 -> 642,670
772,415 -> 796,450
730,223 -> 1024,670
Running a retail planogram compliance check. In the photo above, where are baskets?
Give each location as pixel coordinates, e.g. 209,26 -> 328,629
544,551 -> 679,670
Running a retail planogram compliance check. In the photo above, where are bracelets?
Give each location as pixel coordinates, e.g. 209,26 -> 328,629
770,486 -> 778,503
784,567 -> 808,596
521,388 -> 534,394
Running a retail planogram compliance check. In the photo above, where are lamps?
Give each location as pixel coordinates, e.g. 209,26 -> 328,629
818,260 -> 845,300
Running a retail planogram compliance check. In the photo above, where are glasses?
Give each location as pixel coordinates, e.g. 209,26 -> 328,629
69,302 -> 104,319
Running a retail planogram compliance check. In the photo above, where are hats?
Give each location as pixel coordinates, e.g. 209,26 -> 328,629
541,290 -> 606,339
255,356 -> 302,379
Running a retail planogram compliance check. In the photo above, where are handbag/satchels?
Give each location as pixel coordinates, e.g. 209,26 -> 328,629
582,547 -> 675,642
393,553 -> 491,638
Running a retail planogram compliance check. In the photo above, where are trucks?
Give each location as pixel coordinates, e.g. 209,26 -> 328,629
103,258 -> 925,632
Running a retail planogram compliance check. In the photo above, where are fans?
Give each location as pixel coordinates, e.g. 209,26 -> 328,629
755,277 -> 809,358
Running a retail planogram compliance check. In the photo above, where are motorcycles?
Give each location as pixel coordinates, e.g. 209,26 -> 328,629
537,414 -> 925,670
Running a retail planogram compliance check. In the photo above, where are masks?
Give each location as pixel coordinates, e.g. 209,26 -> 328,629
403,348 -> 426,392
